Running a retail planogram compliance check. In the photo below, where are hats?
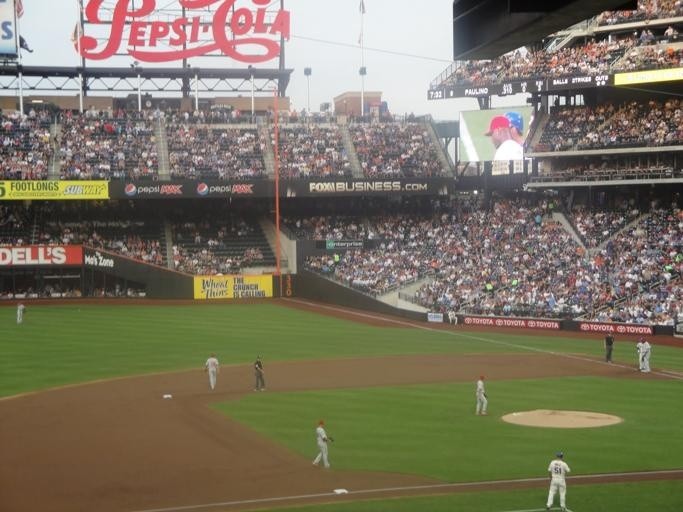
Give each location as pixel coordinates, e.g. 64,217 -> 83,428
484,111 -> 524,136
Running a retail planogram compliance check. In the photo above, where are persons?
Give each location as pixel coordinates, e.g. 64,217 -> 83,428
474,375 -> 488,415
635,338 -> 645,370
202,353 -> 220,389
639,338 -> 651,373
439,1 -> 683,88
544,452 -> 571,512
252,354 -> 266,392
537,95 -> 682,181
1,101 -> 448,182
311,420 -> 333,468
486,111 -> 532,175
603,330 -> 616,363
283,192 -> 683,335
16,302 -> 26,323
2,208 -> 264,299
